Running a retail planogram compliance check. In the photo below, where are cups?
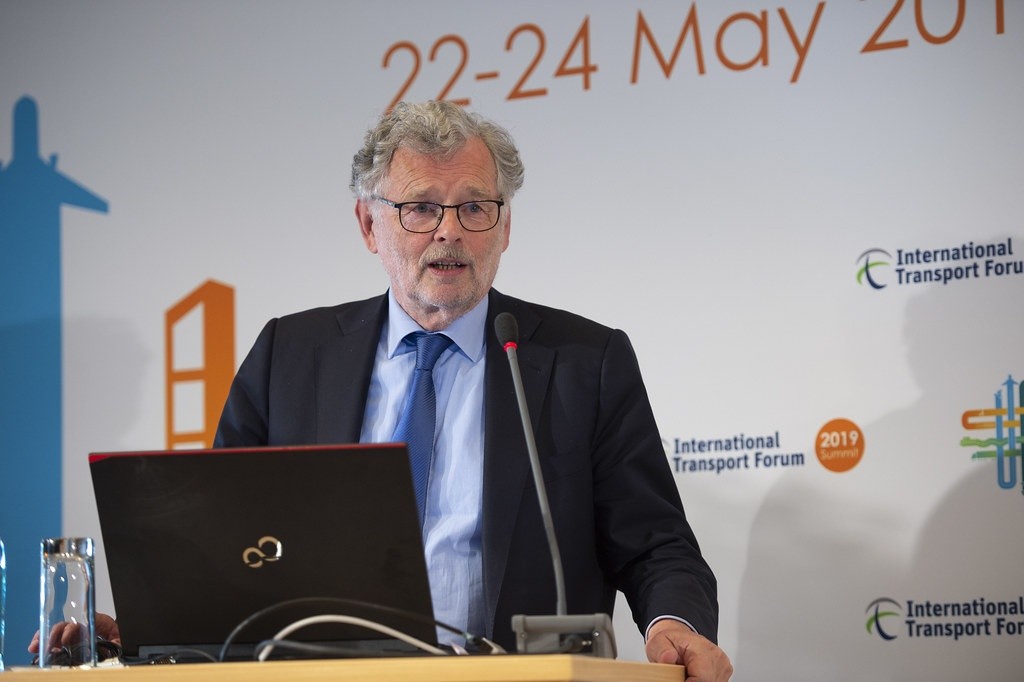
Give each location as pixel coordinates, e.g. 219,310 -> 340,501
39,537 -> 98,669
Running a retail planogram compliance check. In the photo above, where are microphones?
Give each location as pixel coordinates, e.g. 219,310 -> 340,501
494,310 -> 618,660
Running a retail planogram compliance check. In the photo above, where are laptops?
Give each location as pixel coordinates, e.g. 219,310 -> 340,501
87,440 -> 490,667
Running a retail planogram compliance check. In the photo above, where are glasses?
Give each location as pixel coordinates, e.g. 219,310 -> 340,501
370,193 -> 506,234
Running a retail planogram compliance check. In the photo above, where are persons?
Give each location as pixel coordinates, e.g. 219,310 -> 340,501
28,99 -> 733,682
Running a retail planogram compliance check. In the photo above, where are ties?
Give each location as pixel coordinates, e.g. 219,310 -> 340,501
392,330 -> 452,538
